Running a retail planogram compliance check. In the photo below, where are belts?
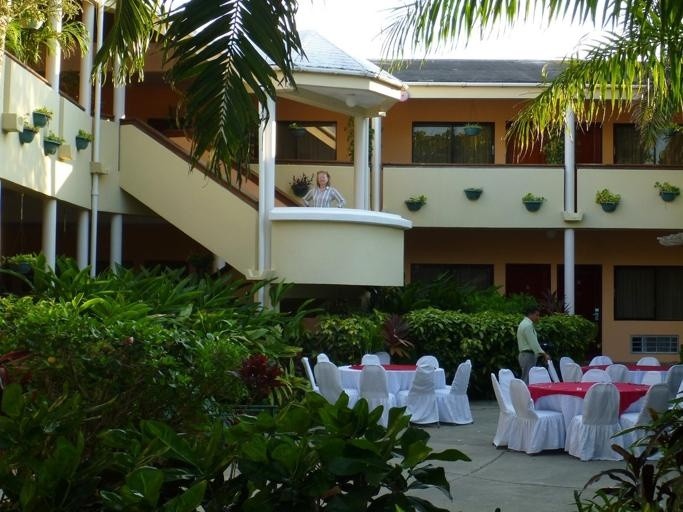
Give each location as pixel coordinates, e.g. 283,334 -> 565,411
521,349 -> 534,354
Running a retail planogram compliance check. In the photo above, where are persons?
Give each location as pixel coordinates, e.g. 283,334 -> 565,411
518,307 -> 555,384
301,170 -> 345,209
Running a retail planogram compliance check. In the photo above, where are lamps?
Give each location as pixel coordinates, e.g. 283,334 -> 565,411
344,94 -> 358,107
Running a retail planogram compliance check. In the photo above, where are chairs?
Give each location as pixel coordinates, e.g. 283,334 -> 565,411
491,355 -> 683,460
300,351 -> 473,429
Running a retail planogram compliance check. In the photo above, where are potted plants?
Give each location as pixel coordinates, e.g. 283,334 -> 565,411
76,129 -> 94,150
405,194 -> 427,210
19,118 -> 39,143
290,172 -> 315,196
464,188 -> 482,200
44,130 -> 65,156
522,193 -> 544,211
595,189 -> 621,212
34,107 -> 53,128
654,181 -> 680,202
